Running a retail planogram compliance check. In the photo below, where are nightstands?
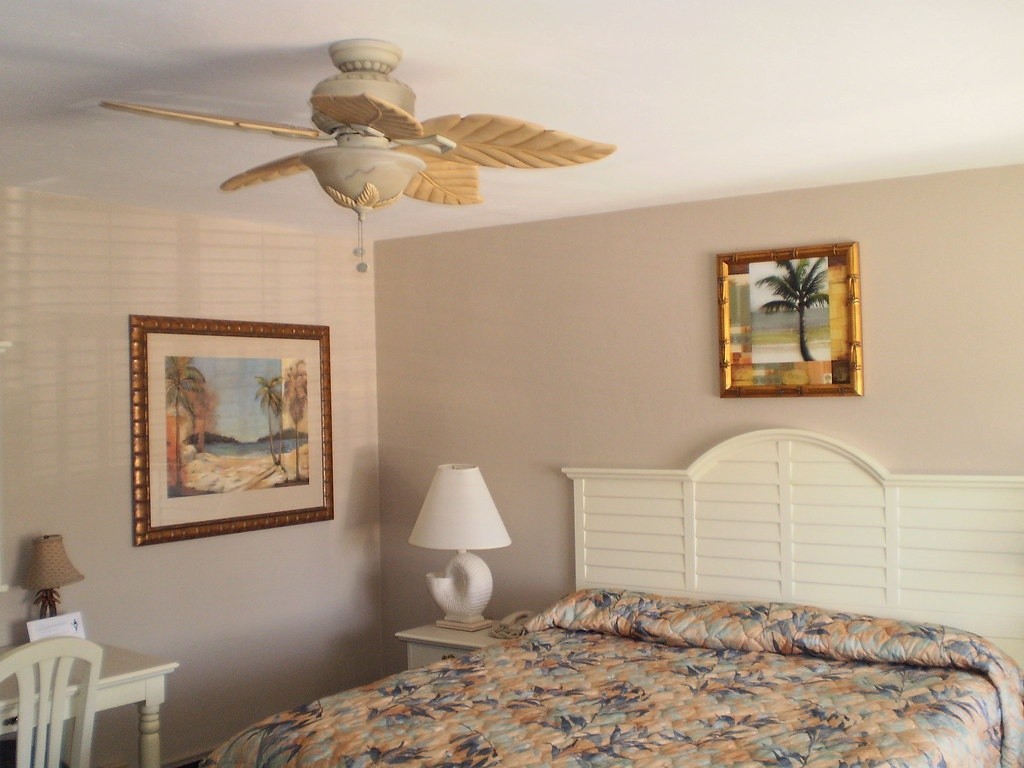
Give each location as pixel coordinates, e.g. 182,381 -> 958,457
395,618 -> 505,670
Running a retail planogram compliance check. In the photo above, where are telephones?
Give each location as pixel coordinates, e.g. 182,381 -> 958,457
499,610 -> 538,633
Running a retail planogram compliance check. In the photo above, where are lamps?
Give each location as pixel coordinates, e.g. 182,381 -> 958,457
407,462 -> 512,632
21,534 -> 85,620
299,133 -> 427,274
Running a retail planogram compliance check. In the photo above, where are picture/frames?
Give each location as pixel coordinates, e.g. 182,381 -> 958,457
129,314 -> 335,547
717,240 -> 865,398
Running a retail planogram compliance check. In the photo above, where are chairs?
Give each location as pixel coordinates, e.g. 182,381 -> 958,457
0,635 -> 103,768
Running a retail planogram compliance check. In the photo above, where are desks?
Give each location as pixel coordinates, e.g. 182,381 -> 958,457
0,637 -> 179,768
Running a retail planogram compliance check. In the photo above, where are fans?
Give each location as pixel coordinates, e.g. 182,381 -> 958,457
98,38 -> 620,205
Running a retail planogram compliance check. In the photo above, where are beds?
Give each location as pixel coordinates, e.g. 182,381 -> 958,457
198,427 -> 1024,768
